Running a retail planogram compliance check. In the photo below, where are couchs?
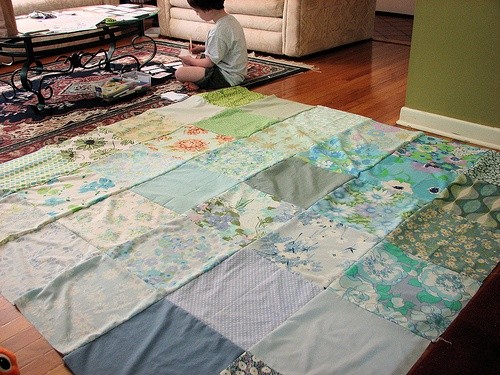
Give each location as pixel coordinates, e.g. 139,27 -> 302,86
0,1 -> 121,53
157,0 -> 377,58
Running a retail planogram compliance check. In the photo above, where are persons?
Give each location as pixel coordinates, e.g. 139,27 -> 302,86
175,0 -> 247,89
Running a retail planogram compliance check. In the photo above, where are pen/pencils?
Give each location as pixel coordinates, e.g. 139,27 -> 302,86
190,36 -> 192,55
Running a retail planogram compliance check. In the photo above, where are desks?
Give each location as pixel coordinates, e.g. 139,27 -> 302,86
1,3 -> 162,112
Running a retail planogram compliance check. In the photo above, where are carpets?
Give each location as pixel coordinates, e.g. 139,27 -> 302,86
0,39 -> 315,163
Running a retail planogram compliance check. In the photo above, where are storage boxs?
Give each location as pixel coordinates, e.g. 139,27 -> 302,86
99,71 -> 151,102
